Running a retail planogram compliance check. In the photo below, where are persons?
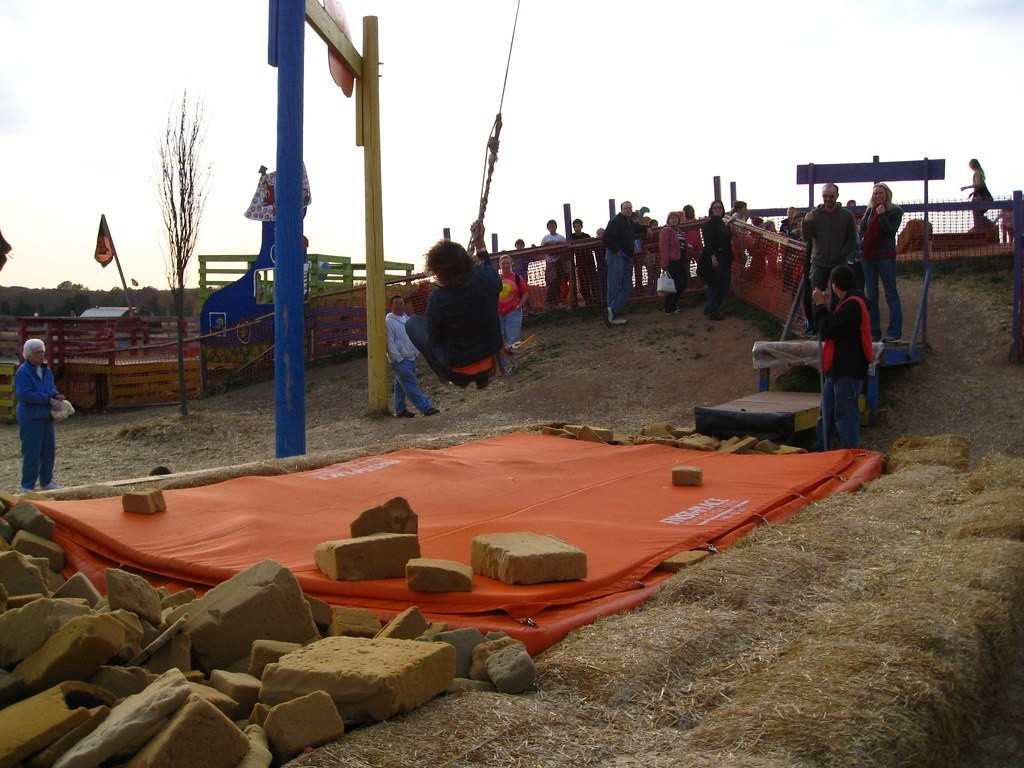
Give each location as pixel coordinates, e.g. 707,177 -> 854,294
779,183 -> 903,344
993,199 -> 1015,244
572,219 -> 602,308
597,228 -> 604,238
726,201 -> 750,282
498,254 -> 529,375
660,211 -> 693,315
812,264 -> 874,452
515,239 -> 525,250
385,295 -> 440,418
645,220 -> 661,290
747,214 -> 779,286
961,159 -> 995,232
603,201 -> 653,328
531,220 -> 567,310
680,205 -> 703,293
14,339 -> 65,494
629,212 -> 649,288
702,200 -> 735,321
405,220 -> 503,388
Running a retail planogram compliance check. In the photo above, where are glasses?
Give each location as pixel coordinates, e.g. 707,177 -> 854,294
713,206 -> 722,210
823,194 -> 834,199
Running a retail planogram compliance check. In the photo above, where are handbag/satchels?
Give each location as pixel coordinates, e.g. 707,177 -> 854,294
656,270 -> 677,297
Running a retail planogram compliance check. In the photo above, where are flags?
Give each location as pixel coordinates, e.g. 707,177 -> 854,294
94,214 -> 115,268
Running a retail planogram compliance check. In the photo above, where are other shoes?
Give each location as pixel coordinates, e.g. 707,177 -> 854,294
21,488 -> 32,493
811,335 -> 818,340
425,407 -> 440,416
397,409 -> 415,418
882,336 -> 902,343
612,318 -> 628,325
663,307 -> 681,315
710,313 -> 725,321
604,307 -> 614,327
40,482 -> 62,490
505,368 -> 512,375
703,310 -> 707,315
872,336 -> 881,342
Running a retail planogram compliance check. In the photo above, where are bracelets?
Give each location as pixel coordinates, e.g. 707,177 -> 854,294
712,256 -> 716,260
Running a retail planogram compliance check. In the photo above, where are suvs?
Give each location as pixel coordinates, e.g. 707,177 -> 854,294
74,305 -> 161,333
